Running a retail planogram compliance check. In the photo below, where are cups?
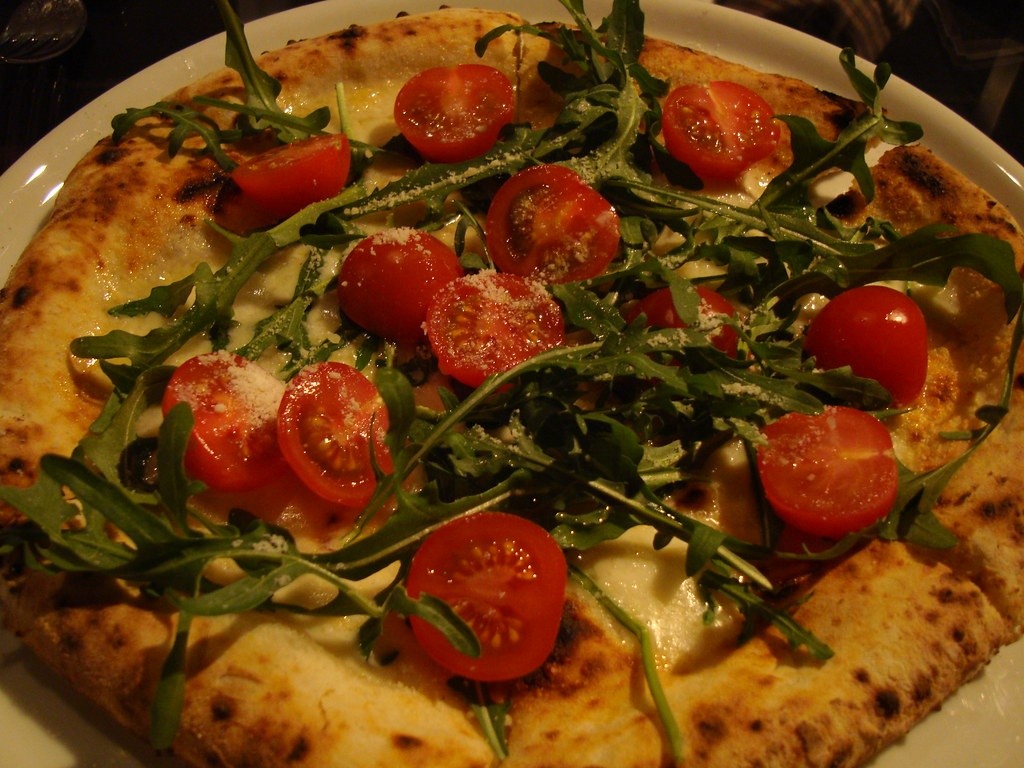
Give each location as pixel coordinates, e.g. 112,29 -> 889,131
0,1 -> 86,64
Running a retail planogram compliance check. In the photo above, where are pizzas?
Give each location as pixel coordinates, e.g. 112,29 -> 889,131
0,0 -> 1024,768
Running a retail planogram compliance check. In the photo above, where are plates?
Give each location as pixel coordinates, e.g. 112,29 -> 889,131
0,1 -> 1024,768
